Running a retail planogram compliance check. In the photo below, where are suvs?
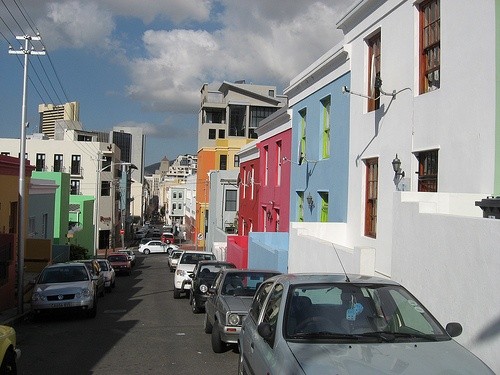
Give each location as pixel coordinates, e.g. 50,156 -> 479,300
161,233 -> 175,244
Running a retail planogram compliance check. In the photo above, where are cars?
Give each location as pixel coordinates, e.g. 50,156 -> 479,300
90,259 -> 117,293
204,270 -> 299,354
27,262 -> 97,318
237,273 -> 496,375
189,261 -> 238,313
70,260 -> 106,299
117,250 -> 136,266
138,240 -> 180,255
168,250 -> 183,273
0,325 -> 18,375
172,251 -> 217,299
131,221 -> 173,239
107,253 -> 132,276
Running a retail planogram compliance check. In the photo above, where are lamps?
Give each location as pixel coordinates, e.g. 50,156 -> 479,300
306,191 -> 316,210
390,152 -> 405,180
267,208 -> 274,222
243,218 -> 248,228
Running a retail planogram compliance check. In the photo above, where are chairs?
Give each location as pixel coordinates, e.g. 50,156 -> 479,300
256,282 -> 262,289
229,280 -> 242,294
202,268 -> 210,272
344,298 -> 386,332
183,255 -> 193,262
292,295 -> 315,326
340,285 -> 364,307
